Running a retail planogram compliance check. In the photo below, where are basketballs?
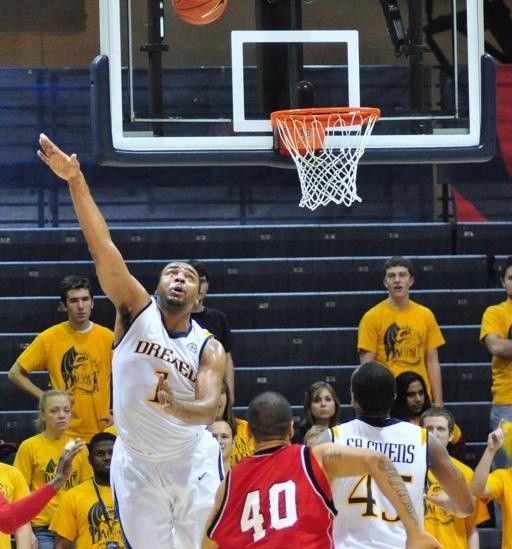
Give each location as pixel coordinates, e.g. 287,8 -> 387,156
172,0 -> 227,25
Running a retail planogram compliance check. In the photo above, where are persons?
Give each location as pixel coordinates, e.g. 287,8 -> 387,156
6,274 -> 115,451
479,255 -> 511,428
37,132 -> 228,548
356,256 -> 446,407
1,364 -> 511,548
189,260 -> 236,404
